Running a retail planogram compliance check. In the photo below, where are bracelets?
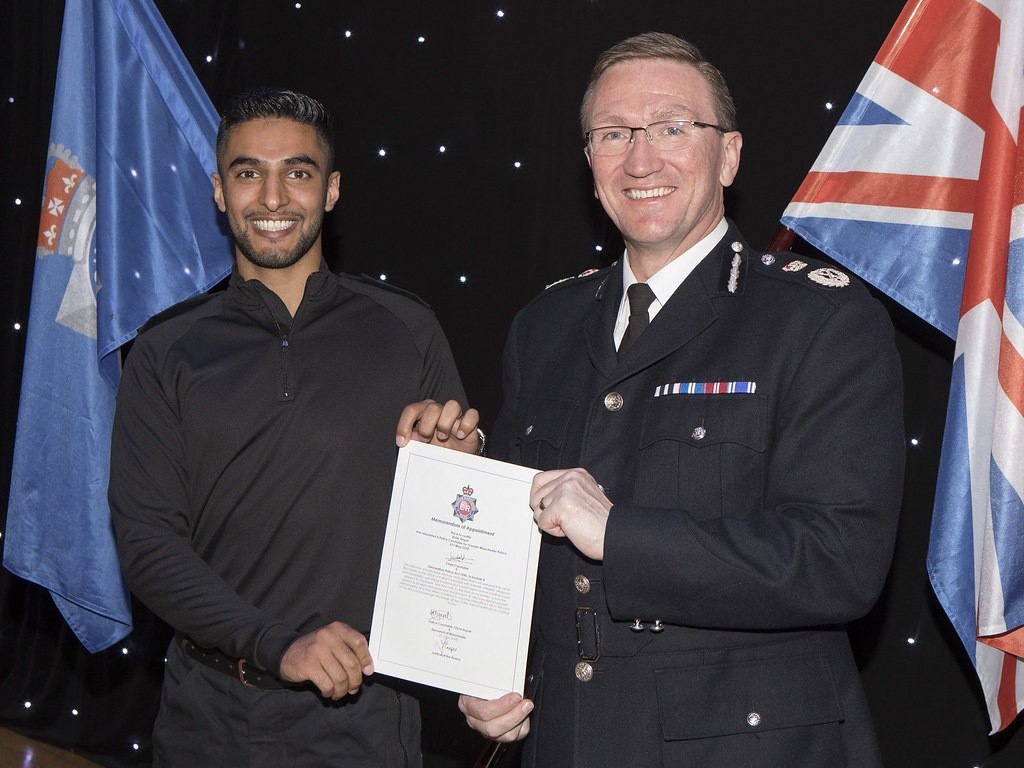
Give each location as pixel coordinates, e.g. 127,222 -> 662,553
475,428 -> 486,457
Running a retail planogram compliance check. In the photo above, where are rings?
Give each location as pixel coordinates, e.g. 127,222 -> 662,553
539,498 -> 546,510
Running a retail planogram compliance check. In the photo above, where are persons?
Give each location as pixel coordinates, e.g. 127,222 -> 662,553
107,88 -> 479,768
457,32 -> 905,768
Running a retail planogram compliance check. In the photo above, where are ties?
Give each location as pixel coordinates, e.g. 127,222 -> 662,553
616,283 -> 657,366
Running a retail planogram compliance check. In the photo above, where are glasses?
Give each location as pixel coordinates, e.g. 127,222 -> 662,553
586,120 -> 728,156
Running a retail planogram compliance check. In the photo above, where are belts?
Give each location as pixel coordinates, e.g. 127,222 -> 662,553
175,629 -> 287,690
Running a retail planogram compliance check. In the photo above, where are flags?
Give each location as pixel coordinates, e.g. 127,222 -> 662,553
1,0 -> 244,653
781,0 -> 1024,735
